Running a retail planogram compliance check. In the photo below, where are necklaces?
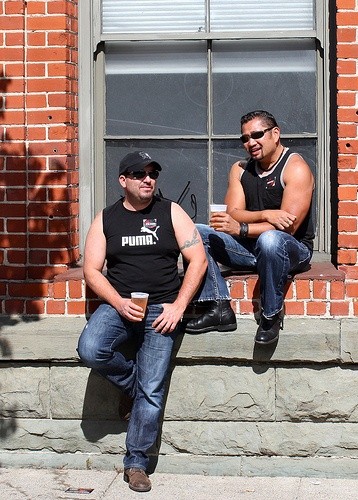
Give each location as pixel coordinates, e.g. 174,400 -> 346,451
258,148 -> 284,172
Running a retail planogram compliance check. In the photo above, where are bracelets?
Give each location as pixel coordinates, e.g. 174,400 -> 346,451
239,223 -> 248,237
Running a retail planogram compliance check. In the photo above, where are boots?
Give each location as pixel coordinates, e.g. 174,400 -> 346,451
255,305 -> 285,344
181,301 -> 236,331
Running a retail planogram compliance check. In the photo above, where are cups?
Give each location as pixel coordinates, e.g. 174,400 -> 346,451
210,205 -> 227,228
131,292 -> 149,318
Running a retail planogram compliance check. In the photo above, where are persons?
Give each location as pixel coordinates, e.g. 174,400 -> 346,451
178,110 -> 315,345
76,151 -> 208,492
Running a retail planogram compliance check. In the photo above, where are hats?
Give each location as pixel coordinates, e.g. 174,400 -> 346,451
119,151 -> 162,174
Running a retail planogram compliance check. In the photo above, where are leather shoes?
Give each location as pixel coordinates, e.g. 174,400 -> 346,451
119,393 -> 132,420
124,468 -> 151,492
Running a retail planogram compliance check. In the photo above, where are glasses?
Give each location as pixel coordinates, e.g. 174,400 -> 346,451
239,127 -> 273,143
127,170 -> 159,179
